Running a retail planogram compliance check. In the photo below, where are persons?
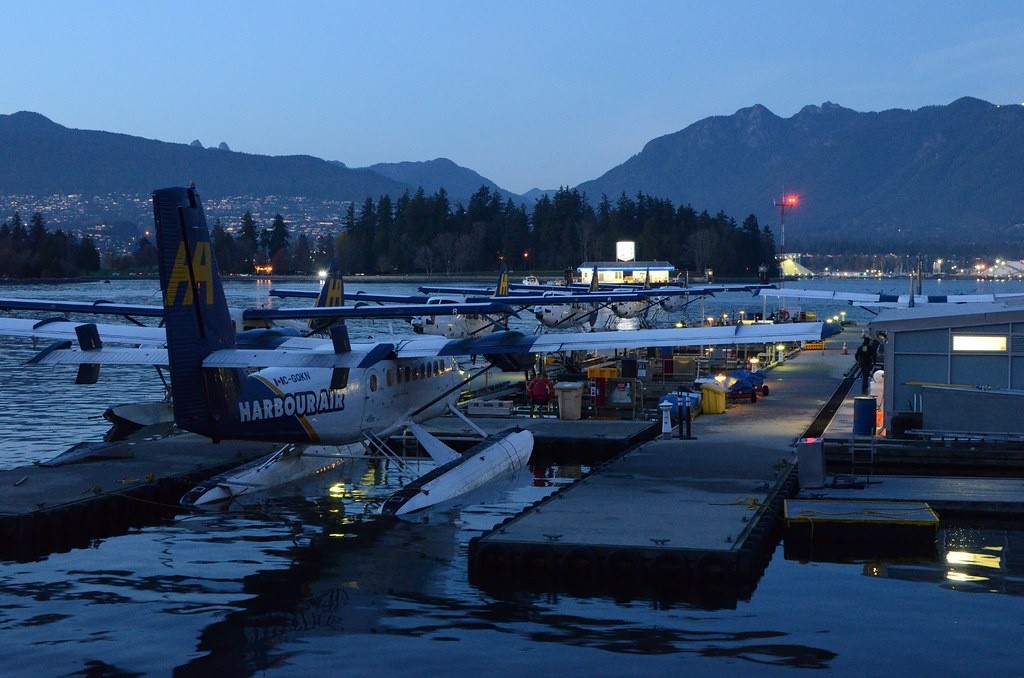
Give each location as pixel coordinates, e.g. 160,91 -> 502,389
855,335 -> 878,394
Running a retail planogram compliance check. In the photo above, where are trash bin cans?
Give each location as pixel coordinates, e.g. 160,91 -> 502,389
700,384 -> 726,414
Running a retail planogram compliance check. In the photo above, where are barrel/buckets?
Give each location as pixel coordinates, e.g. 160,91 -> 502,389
870,382 -> 883,410
853,397 -> 876,435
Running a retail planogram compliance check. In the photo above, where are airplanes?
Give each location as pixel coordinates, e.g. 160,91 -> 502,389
0,181 -> 1024,526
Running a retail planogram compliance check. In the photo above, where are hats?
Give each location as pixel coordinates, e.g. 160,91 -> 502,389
865,337 -> 871,342
